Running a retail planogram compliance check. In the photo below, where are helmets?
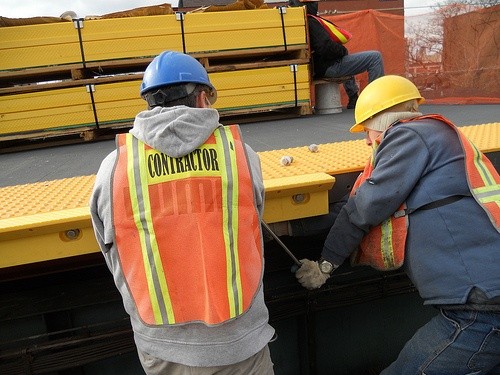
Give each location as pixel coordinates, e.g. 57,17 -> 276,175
139,50 -> 218,105
349,75 -> 426,133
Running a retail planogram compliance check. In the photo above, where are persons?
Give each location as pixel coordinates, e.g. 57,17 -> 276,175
91,51 -> 276,375
295,75 -> 500,375
290,0 -> 385,109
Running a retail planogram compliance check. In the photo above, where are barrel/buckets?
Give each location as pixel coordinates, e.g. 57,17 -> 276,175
315,82 -> 343,114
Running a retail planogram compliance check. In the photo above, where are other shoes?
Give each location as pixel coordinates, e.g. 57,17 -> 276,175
346,95 -> 359,109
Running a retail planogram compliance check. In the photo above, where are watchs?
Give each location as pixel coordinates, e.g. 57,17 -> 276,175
319,256 -> 335,276
